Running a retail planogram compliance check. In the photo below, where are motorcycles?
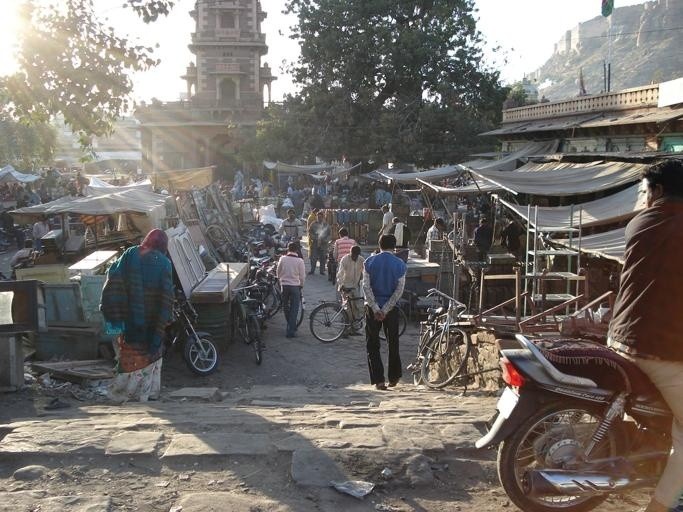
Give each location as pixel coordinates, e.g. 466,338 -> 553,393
165,284 -> 220,377
467,332 -> 671,512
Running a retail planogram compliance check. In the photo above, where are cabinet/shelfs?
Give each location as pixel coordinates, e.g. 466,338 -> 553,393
523,203 -> 583,328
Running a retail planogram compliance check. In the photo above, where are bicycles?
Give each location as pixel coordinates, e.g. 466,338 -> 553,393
405,286 -> 471,391
307,284 -> 408,344
222,220 -> 305,331
226,282 -> 266,366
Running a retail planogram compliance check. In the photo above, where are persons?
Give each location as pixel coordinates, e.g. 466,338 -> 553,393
606,158 -> 682,511
0,161 -> 528,404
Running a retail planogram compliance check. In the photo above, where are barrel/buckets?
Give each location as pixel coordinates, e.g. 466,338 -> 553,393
194,303 -> 231,354
